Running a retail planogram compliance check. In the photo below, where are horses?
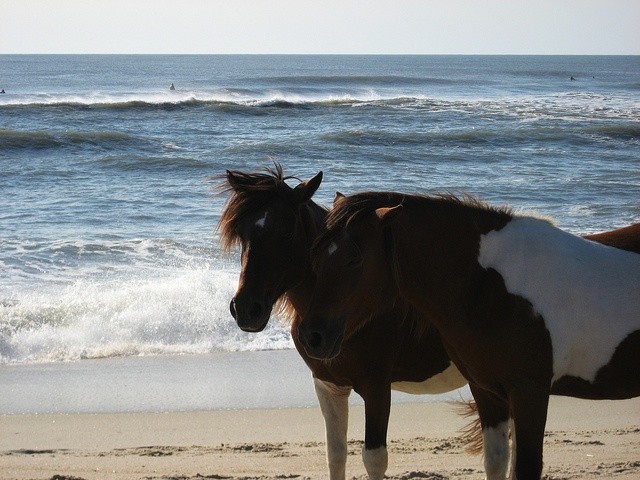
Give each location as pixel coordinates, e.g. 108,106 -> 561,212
211,162 -> 640,480
298,191 -> 640,480
